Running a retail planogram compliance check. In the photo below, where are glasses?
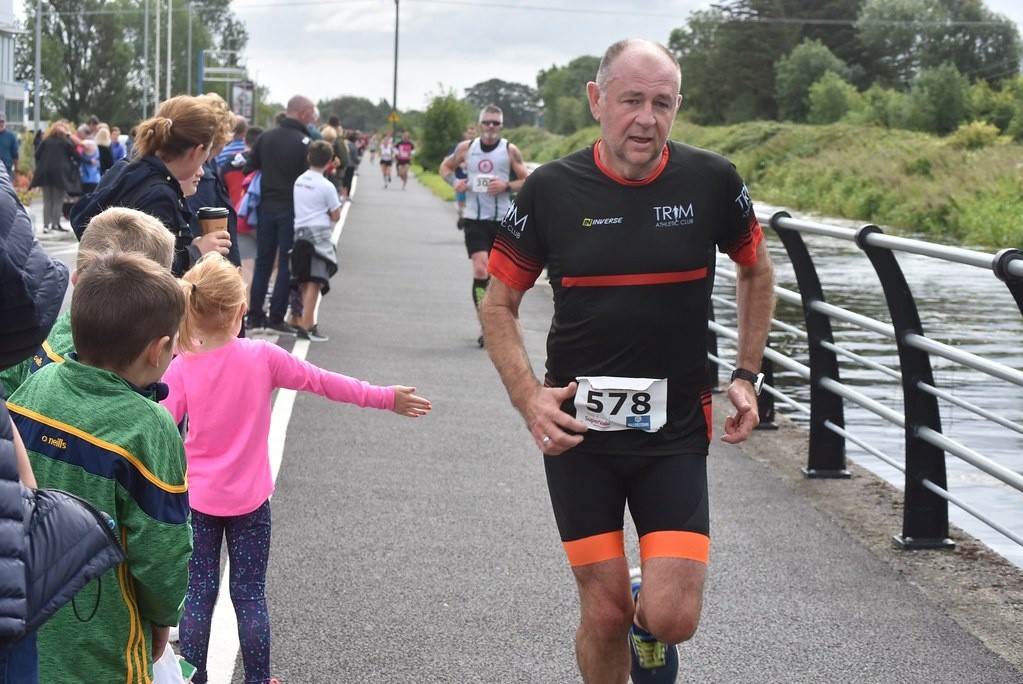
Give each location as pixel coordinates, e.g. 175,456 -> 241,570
481,120 -> 502,127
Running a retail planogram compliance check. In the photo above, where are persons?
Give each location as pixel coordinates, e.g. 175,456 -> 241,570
0,112 -> 21,188
440,106 -> 528,347
74,94 -> 232,271
0,205 -> 175,399
178,92 -> 368,342
477,39 -> 776,684
443,125 -> 476,219
0,158 -> 127,684
28,115 -> 139,233
378,136 -> 395,189
158,256 -> 433,684
393,132 -> 415,189
6,247 -> 193,684
369,129 -> 404,162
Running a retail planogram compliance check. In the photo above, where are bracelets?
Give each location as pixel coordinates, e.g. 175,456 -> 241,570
66,132 -> 71,137
15,168 -> 20,172
505,182 -> 511,193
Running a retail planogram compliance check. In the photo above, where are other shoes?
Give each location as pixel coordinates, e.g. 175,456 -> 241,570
458,216 -> 463,229
51,225 -> 69,234
43,225 -> 49,233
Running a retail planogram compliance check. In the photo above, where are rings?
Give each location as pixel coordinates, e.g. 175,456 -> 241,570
543,436 -> 551,445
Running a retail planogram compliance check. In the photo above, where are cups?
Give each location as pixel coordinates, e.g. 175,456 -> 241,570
196,206 -> 229,238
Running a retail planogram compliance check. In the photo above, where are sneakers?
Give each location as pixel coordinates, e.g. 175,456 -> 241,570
266,319 -> 299,336
478,335 -> 485,347
245,312 -> 267,332
628,568 -> 680,684
297,324 -> 330,342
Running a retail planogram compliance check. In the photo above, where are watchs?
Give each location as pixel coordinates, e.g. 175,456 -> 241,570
731,369 -> 765,397
